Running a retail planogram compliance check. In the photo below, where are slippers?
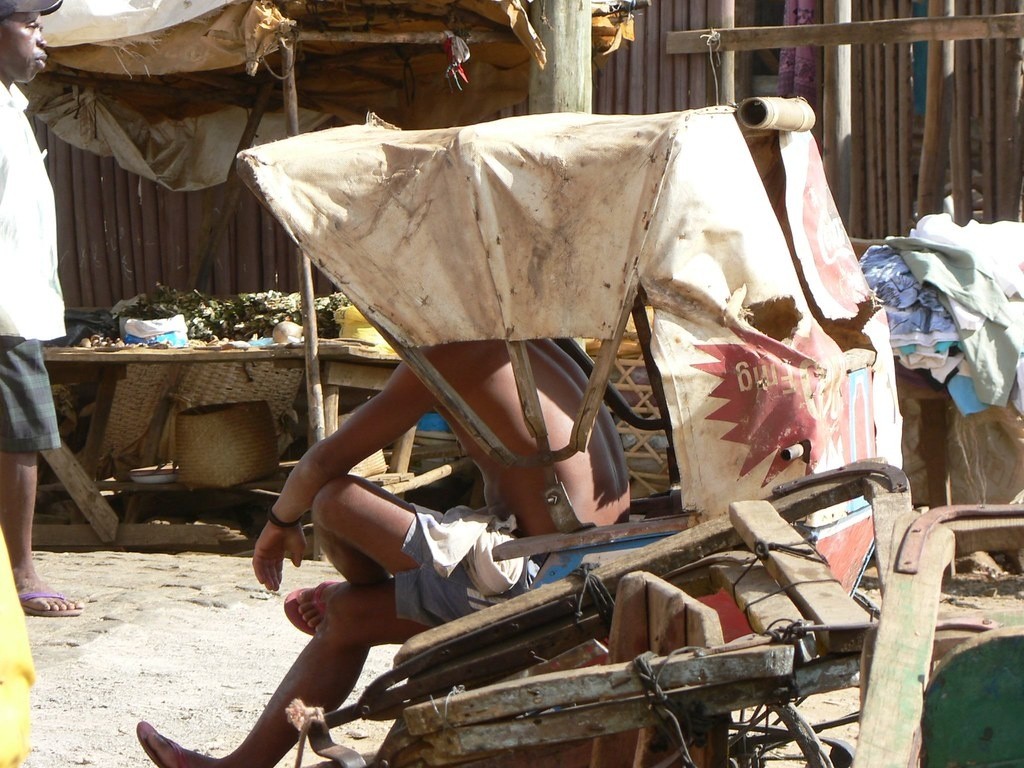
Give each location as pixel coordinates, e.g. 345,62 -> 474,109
19,591 -> 85,617
136,720 -> 188,768
283,579 -> 342,636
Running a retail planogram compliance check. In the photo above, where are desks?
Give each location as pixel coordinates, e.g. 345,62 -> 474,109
29,345 -> 418,562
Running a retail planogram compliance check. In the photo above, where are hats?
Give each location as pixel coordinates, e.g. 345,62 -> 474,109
0,0 -> 64,20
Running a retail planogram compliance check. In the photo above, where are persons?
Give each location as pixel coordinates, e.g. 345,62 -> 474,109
136,339 -> 630,768
0,0 -> 84,617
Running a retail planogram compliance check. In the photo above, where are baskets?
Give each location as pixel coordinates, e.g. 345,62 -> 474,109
175,400 -> 279,493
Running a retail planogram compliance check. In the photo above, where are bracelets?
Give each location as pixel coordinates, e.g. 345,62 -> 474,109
267,503 -> 303,527
